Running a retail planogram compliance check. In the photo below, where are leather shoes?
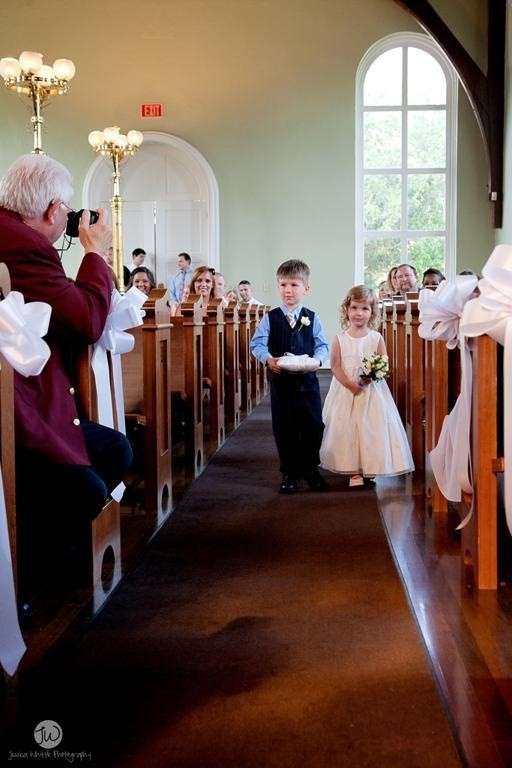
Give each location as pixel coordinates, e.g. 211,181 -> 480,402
349,475 -> 364,490
310,478 -> 331,491
278,478 -> 302,494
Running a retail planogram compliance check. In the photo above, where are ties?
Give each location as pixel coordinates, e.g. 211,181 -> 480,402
288,313 -> 296,328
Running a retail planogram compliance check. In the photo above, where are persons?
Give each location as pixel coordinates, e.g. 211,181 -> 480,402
96,245 -> 266,309
315,284 -> 416,488
0,150 -> 137,624
377,262 -> 485,298
223,258 -> 331,478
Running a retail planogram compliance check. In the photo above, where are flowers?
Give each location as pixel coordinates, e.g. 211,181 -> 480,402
356,354 -> 390,390
298,314 -> 310,332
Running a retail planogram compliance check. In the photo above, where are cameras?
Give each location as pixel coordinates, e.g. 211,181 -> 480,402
66,208 -> 98,237
